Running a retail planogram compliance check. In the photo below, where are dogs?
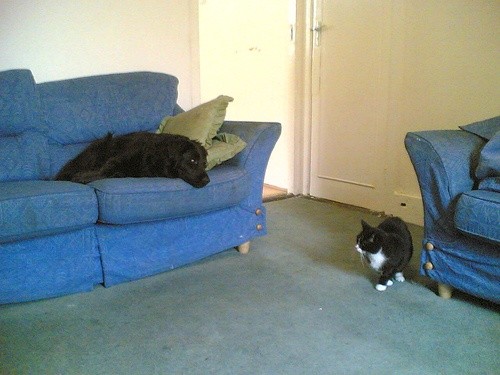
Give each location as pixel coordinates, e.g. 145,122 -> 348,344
50,130 -> 211,190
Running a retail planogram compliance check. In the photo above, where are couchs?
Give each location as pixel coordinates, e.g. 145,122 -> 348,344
0,68 -> 282,305
404,115 -> 500,304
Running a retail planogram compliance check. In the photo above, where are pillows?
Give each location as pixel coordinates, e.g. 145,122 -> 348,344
205,133 -> 247,171
156,95 -> 234,151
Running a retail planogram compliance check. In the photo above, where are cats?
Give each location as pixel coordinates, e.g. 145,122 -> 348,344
352,215 -> 414,292
